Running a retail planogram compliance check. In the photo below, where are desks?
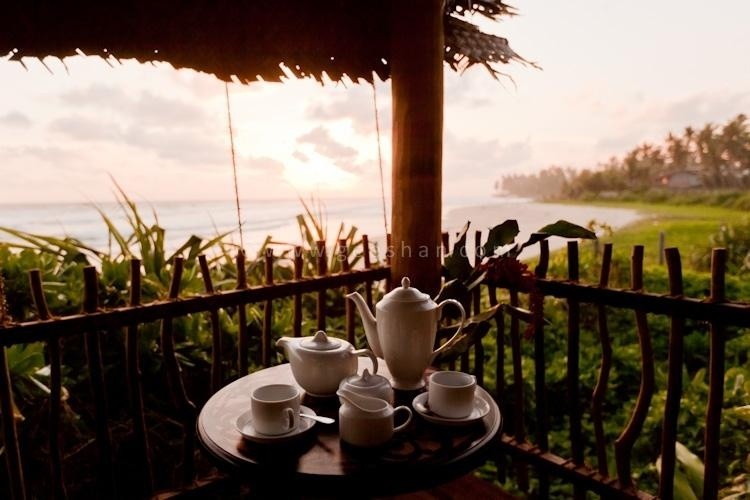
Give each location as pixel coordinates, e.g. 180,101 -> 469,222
197,356 -> 503,500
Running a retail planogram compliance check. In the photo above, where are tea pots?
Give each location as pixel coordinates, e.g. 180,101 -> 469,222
339,369 -> 398,407
345,274 -> 467,391
330,384 -> 414,444
276,331 -> 381,397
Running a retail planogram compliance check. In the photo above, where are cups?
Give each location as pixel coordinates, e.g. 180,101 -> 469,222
425,366 -> 478,419
250,381 -> 299,435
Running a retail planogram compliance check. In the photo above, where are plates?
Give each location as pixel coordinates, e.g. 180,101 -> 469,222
412,390 -> 491,424
233,406 -> 319,442
